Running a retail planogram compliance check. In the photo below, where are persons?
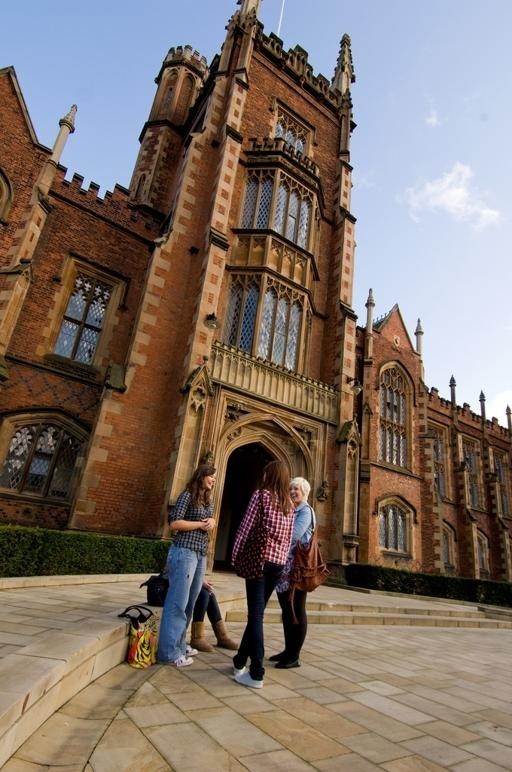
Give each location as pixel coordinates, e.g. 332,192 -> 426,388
190,580 -> 238,652
231,459 -> 296,690
155,465 -> 217,669
269,476 -> 317,670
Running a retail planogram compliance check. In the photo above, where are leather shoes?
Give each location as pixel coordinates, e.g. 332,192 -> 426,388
269,652 -> 301,668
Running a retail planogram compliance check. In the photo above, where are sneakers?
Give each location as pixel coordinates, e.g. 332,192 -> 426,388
183,645 -> 198,657
156,655 -> 194,667
233,663 -> 264,689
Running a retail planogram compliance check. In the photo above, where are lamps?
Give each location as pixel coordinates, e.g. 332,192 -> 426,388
204,312 -> 220,329
346,375 -> 364,396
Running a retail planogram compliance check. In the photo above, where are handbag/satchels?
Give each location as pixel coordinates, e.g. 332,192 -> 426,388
139,576 -> 168,606
127,612 -> 159,668
234,526 -> 270,580
290,531 -> 331,592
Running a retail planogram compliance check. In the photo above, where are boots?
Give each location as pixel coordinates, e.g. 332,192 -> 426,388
212,619 -> 241,650
190,622 -> 214,652
